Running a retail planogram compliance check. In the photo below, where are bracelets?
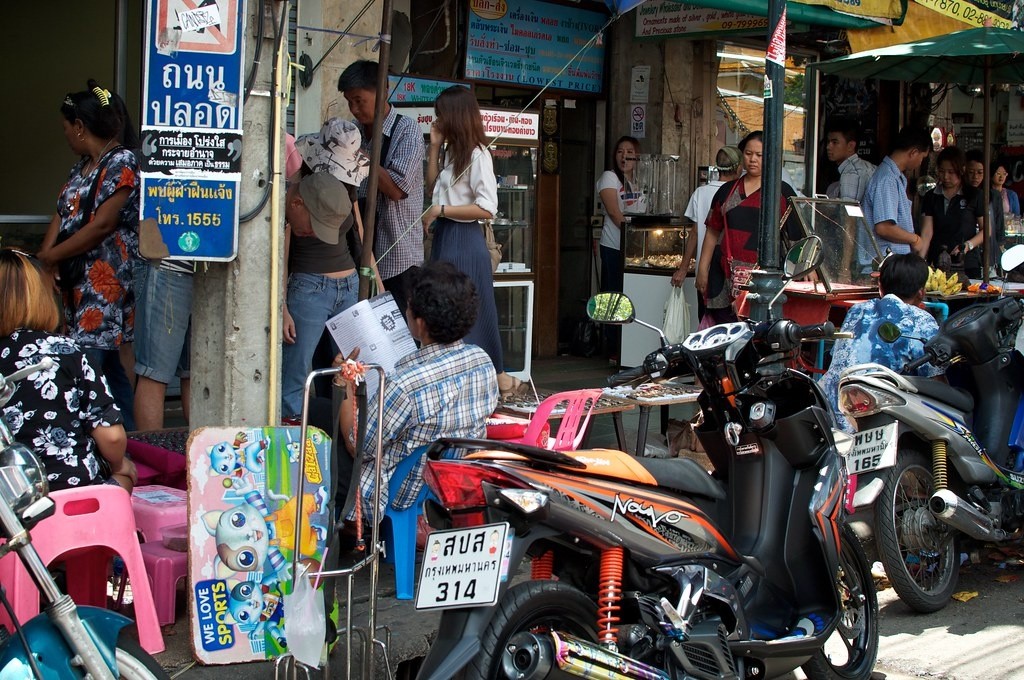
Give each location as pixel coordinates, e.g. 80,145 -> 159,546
439,205 -> 446,220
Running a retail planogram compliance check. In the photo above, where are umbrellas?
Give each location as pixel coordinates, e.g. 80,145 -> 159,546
811,18 -> 1024,293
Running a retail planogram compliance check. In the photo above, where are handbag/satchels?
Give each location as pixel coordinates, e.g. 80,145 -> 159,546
662,285 -> 691,345
730,259 -> 760,297
485,222 -> 502,273
56,231 -> 86,291
939,246 -> 966,290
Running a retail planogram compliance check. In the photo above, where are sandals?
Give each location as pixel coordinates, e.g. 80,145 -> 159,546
500,376 -> 529,403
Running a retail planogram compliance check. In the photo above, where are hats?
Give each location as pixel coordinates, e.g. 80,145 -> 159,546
297,173 -> 353,245
294,118 -> 372,186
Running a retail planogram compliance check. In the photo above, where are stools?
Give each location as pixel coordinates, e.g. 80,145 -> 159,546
131,484 -> 188,542
0,485 -> 168,655
115,541 -> 187,625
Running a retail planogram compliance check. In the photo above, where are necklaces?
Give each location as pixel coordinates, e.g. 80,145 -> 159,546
74,139 -> 117,201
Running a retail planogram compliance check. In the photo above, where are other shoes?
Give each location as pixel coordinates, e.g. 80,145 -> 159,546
608,356 -> 620,367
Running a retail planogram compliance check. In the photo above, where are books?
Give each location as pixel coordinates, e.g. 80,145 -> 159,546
325,291 -> 417,403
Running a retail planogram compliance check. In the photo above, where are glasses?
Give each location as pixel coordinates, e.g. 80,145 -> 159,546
995,172 -> 1008,177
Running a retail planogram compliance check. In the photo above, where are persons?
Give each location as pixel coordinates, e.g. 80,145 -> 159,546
916,145 -> 991,283
136,119 -> 386,432
0,248 -> 138,498
825,128 -> 881,287
596,135 -> 650,364
697,131 -> 812,328
420,85 -> 505,423
37,80 -> 141,435
962,147 -> 1021,279
335,60 -> 426,335
816,253 -> 943,442
670,146 -> 747,326
336,264 -> 500,565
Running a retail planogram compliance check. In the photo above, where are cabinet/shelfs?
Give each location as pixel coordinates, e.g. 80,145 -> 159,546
491,139 -> 543,279
493,281 -> 535,382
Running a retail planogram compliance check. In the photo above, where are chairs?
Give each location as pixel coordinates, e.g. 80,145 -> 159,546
380,443 -> 457,599
505,387 -> 603,451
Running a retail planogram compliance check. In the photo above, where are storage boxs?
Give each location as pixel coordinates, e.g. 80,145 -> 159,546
618,210 -> 698,275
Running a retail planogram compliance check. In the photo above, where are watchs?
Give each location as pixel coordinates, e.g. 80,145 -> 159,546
965,241 -> 974,252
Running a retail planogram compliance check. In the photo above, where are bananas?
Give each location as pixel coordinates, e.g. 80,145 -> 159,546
923,266 -> 963,295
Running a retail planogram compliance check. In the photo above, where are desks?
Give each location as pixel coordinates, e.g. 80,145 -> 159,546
495,392 -> 636,454
603,384 -> 700,457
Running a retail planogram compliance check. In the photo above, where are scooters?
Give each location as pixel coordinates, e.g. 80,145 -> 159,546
839,242 -> 1024,608
0,357 -> 172,680
414,235 -> 879,680
